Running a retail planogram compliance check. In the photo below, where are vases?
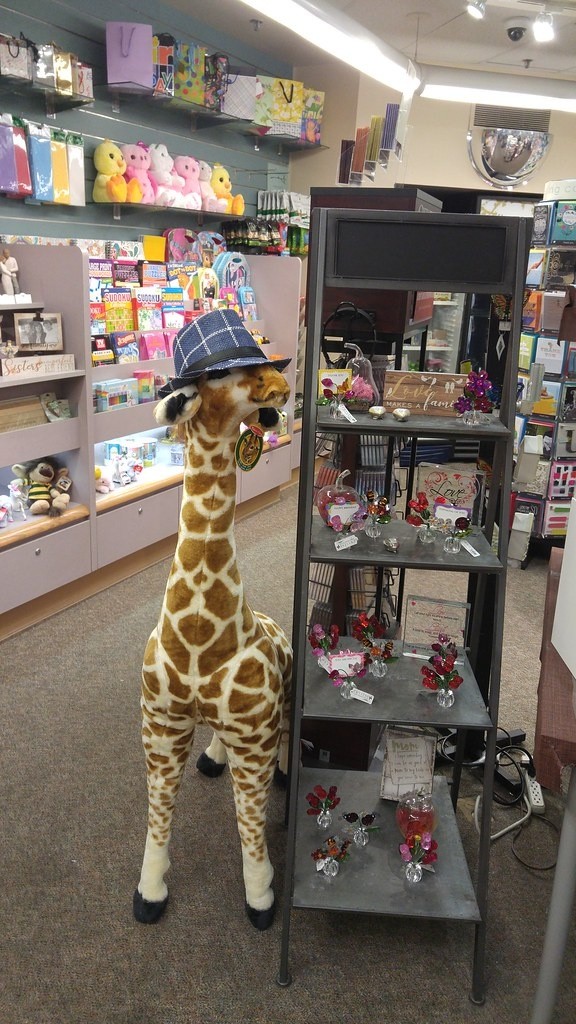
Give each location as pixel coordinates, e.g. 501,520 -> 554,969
436,689 -> 455,706
371,660 -> 388,679
417,525 -> 438,544
405,861 -> 423,882
316,809 -> 333,829
353,828 -> 369,847
462,409 -> 492,426
322,860 -> 340,876
365,519 -> 383,539
339,681 -> 358,700
330,399 -> 348,420
318,652 -> 334,668
363,641 -> 378,655
444,537 -> 461,553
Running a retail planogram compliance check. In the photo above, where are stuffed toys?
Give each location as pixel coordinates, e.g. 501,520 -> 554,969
133,310 -> 293,931
13,456 -> 70,517
94,464 -> 115,494
91,139 -> 245,215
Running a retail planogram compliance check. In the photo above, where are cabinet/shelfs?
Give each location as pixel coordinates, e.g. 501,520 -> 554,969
275,207 -> 533,1008
0,244 -> 308,619
403,293 -> 465,372
0,73 -> 332,227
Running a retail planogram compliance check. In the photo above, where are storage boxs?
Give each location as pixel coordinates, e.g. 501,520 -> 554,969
97,378 -> 139,411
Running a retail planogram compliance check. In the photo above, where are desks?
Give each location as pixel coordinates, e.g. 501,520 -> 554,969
530,546 -> 576,1024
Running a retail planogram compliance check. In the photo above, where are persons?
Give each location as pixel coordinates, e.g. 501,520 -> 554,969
20,321 -> 58,343
0,248 -> 20,295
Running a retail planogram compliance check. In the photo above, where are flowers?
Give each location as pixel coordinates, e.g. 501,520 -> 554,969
398,832 -> 439,864
341,812 -> 382,834
309,837 -> 351,870
310,370 -> 493,692
304,785 -> 340,815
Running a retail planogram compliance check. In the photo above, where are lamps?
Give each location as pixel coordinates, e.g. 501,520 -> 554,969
239,0 -> 576,115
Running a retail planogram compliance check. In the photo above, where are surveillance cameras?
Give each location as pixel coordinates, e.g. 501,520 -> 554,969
504,17 -> 531,41
